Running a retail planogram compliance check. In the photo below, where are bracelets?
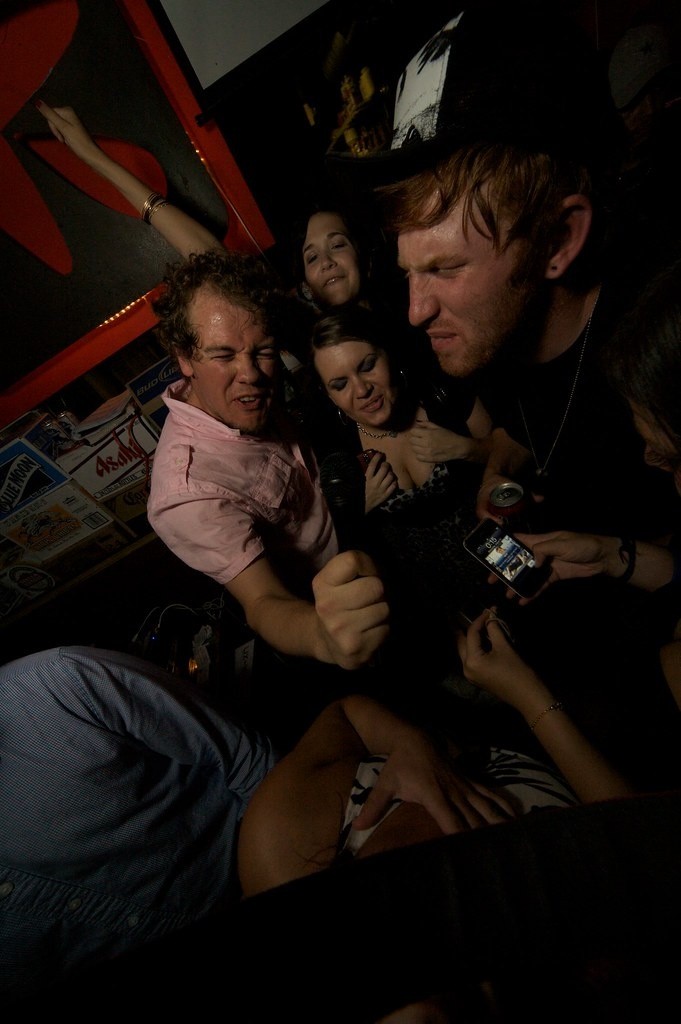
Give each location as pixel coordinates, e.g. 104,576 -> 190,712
141,192 -> 170,224
615,536 -> 637,581
531,701 -> 563,730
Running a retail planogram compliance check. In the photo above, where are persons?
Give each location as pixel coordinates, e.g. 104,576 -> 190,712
0,24 -> 681,1024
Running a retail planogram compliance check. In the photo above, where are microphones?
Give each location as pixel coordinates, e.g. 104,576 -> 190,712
319,452 -> 367,577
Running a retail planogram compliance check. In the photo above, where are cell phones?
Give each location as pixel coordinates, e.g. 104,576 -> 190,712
463,518 -> 554,599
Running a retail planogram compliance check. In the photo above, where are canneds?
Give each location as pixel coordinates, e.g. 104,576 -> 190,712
488,482 -> 530,533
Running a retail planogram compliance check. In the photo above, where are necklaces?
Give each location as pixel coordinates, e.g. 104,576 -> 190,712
506,291 -> 594,479
356,422 -> 398,439
